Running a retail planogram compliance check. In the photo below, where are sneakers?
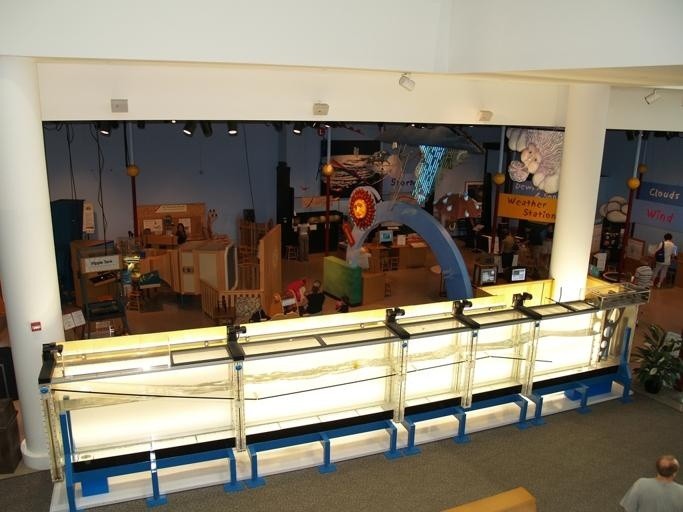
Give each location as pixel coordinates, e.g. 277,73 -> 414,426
656,283 -> 661,288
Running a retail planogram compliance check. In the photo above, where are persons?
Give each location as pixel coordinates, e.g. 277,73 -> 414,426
175,223 -> 187,244
629,256 -> 654,291
335,294 -> 349,313
304,279 -> 325,314
618,454 -> 683,511
543,223 -> 554,269
652,232 -> 677,289
501,234 -> 519,269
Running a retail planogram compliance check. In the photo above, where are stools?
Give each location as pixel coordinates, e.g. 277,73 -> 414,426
285,245 -> 298,262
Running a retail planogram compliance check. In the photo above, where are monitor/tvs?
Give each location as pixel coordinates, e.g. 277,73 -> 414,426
509,266 -> 527,282
375,230 -> 394,244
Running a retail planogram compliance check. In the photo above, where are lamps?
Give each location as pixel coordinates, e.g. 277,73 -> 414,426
93,120 -> 336,138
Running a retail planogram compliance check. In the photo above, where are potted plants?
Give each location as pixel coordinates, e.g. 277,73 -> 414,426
633,320 -> 683,395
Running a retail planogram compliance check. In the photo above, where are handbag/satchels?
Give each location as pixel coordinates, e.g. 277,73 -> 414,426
656,250 -> 664,262
